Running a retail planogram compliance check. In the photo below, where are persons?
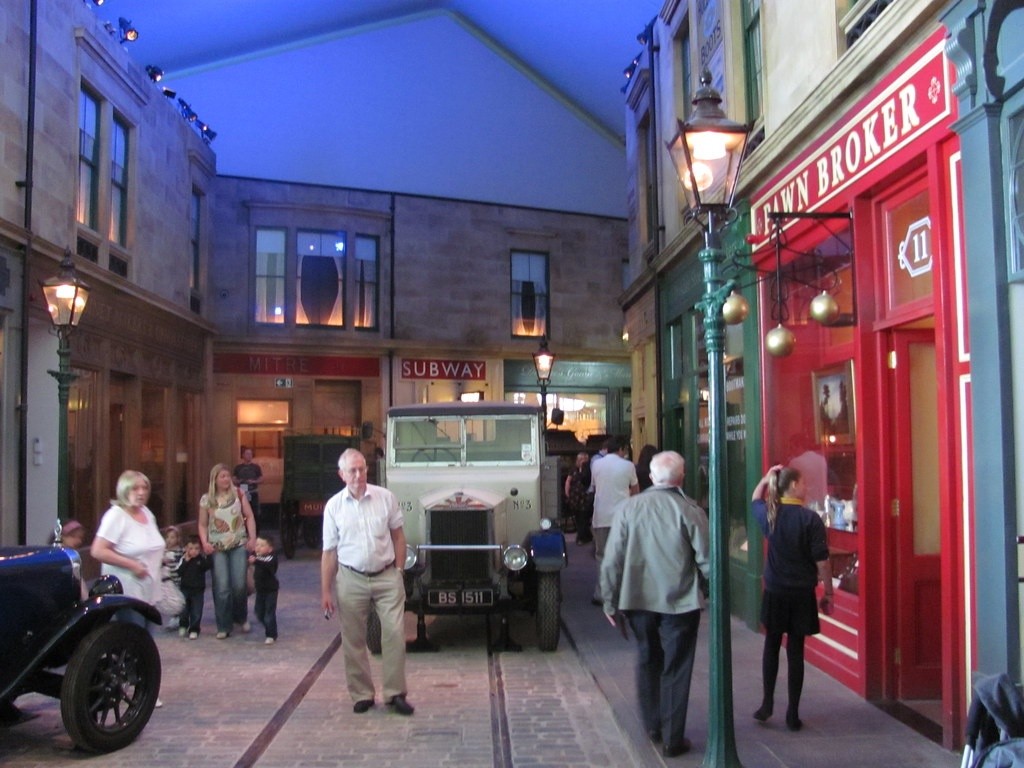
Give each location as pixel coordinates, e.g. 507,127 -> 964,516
163,464 -> 279,643
91,471 -> 166,708
232,449 -> 264,537
49,521 -> 83,579
752,466 -> 835,731
601,456 -> 708,756
565,438 -> 657,605
321,449 -> 414,715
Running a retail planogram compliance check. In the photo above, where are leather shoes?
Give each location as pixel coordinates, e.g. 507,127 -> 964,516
353,699 -> 375,714
392,696 -> 414,715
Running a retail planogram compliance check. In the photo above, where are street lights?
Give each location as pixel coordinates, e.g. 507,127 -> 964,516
533,333 -> 557,438
37,241 -> 91,530
663,65 -> 758,767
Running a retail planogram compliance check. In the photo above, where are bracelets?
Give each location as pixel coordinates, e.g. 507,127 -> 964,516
398,567 -> 404,572
825,592 -> 833,595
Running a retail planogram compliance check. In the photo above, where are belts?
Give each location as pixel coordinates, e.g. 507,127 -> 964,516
340,560 -> 395,577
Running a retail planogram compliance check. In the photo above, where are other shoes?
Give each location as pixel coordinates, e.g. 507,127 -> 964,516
178,627 -> 187,638
753,704 -> 773,721
786,712 -> 802,733
650,731 -> 662,742
662,737 -> 691,758
189,632 -> 198,640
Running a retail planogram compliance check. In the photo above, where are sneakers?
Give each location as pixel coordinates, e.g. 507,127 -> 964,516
216,632 -> 228,639
163,617 -> 181,631
264,637 -> 274,645
242,622 -> 251,632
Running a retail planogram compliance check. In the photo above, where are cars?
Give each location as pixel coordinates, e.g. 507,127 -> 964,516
0,520 -> 164,757
364,400 -> 572,654
